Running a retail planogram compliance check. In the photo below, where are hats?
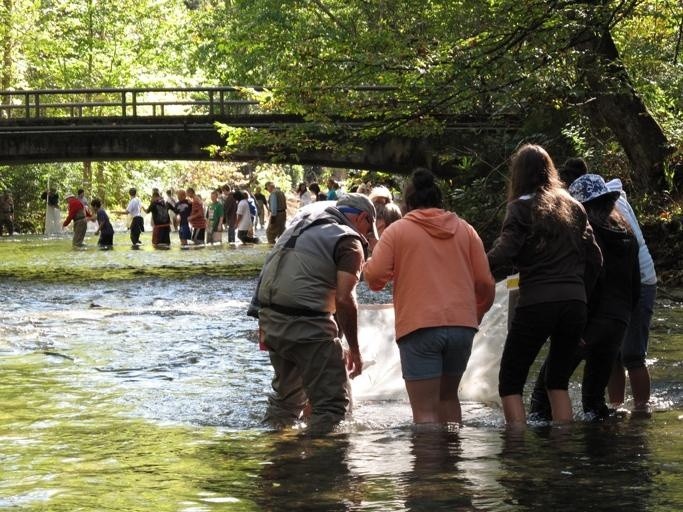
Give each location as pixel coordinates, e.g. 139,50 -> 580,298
369,187 -> 391,202
566,172 -> 621,206
337,193 -> 379,241
63,192 -> 75,201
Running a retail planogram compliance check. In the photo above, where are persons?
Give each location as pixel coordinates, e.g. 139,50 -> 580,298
485,144 -> 604,432
1,183 -> 15,235
245,184 -> 402,433
63,177 -> 378,248
528,155 -> 658,422
362,167 -> 498,430
42,186 -> 62,233
